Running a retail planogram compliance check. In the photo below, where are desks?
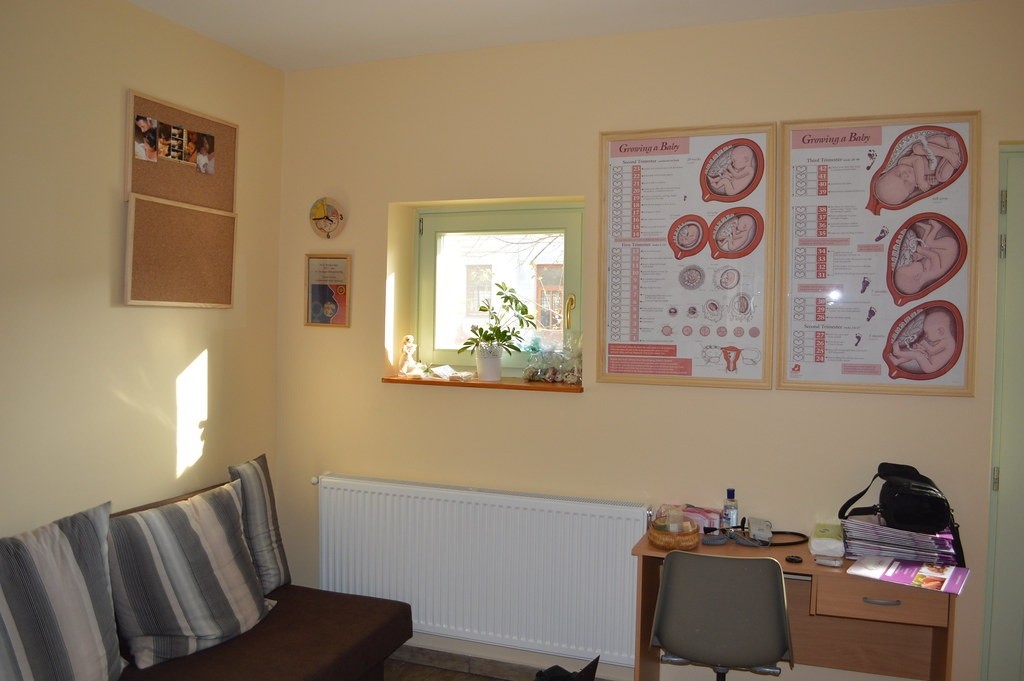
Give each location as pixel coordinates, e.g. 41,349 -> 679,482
631,527 -> 958,681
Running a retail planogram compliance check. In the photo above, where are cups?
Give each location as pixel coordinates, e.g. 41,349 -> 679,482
667,508 -> 683,533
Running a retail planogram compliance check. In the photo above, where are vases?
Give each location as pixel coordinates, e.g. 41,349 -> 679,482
475,345 -> 503,382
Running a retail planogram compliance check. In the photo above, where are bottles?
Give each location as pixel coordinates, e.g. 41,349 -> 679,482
723,488 -> 738,532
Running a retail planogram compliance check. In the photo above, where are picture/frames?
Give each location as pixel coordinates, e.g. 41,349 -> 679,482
304,254 -> 353,328
776,109 -> 981,397
123,193 -> 237,309
125,89 -> 239,213
597,120 -> 778,390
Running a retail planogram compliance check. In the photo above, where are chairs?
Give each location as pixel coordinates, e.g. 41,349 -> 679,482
649,549 -> 793,681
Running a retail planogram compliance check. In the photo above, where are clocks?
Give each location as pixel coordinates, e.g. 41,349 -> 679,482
309,198 -> 346,239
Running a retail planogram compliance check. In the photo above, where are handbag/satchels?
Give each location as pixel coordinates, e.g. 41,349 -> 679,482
838,461 -> 966,568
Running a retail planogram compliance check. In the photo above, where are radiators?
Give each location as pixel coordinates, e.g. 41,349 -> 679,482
312,472 -> 652,668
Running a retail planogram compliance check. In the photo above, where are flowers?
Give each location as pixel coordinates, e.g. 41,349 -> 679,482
458,283 -> 537,356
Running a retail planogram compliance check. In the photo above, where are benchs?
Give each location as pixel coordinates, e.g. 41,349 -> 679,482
109,481 -> 414,681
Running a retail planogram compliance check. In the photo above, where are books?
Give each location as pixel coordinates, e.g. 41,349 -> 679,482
840,515 -> 970,596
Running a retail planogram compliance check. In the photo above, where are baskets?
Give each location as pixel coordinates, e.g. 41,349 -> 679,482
647,515 -> 700,551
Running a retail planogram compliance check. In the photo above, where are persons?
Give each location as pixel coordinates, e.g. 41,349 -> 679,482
135,115 -> 157,162
157,123 -> 184,161
185,131 -> 215,175
399,335 -> 417,376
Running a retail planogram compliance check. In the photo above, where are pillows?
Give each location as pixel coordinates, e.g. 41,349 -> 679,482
107,478 -> 278,668
226,453 -> 291,597
0,500 -> 130,680
